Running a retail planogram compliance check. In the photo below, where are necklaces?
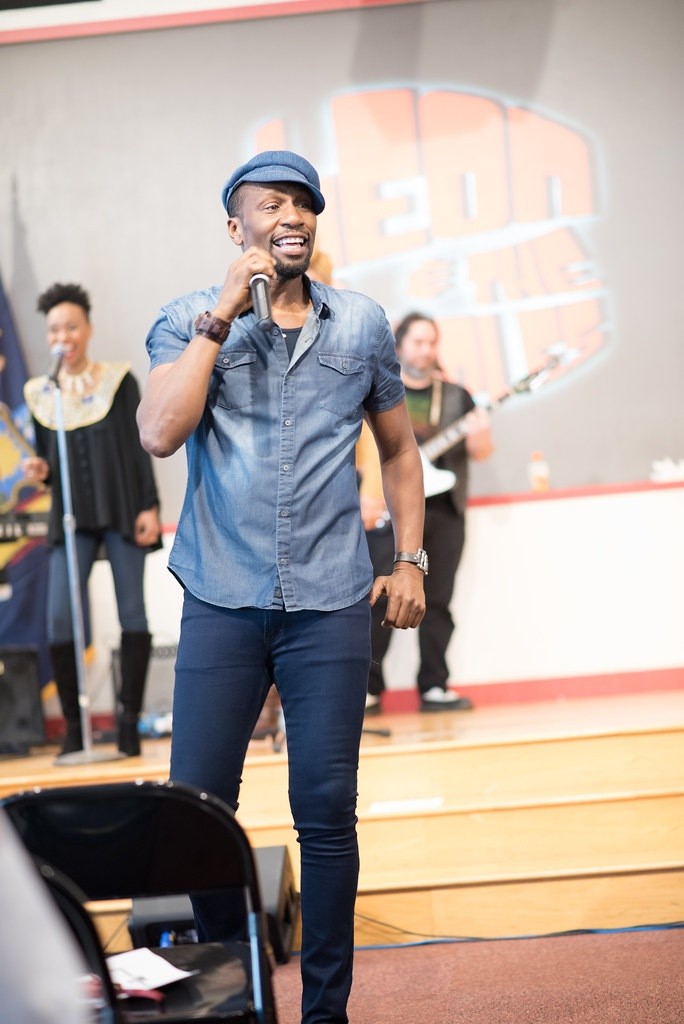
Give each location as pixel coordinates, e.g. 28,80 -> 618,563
61,363 -> 95,396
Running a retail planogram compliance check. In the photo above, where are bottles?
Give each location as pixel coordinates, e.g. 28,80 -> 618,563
138,714 -> 172,739
530,451 -> 548,491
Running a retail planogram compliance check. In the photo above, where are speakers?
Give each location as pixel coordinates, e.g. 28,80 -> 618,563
111,645 -> 175,715
127,845 -> 299,965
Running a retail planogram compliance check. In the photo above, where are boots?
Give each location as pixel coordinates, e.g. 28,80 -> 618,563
118,631 -> 154,756
50,642 -> 84,755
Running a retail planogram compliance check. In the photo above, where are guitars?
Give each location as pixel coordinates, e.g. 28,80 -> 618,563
374,366 -> 550,532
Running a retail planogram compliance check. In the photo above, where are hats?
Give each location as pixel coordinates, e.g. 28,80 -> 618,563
222,151 -> 325,217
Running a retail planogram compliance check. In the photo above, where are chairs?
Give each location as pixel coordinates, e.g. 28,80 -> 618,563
0,777 -> 280,1024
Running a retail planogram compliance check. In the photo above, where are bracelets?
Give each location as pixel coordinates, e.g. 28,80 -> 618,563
192,311 -> 232,347
391,548 -> 431,575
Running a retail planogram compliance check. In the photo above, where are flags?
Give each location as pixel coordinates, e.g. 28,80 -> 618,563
1,280 -> 77,759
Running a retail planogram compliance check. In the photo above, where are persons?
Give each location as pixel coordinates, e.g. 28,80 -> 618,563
360,314 -> 493,716
136,151 -> 430,1024
21,282 -> 164,763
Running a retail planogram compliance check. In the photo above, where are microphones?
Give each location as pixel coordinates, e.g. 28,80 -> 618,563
249,271 -> 274,331
49,343 -> 66,381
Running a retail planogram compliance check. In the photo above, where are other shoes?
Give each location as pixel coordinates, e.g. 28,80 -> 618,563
363,692 -> 380,716
421,686 -> 471,712
